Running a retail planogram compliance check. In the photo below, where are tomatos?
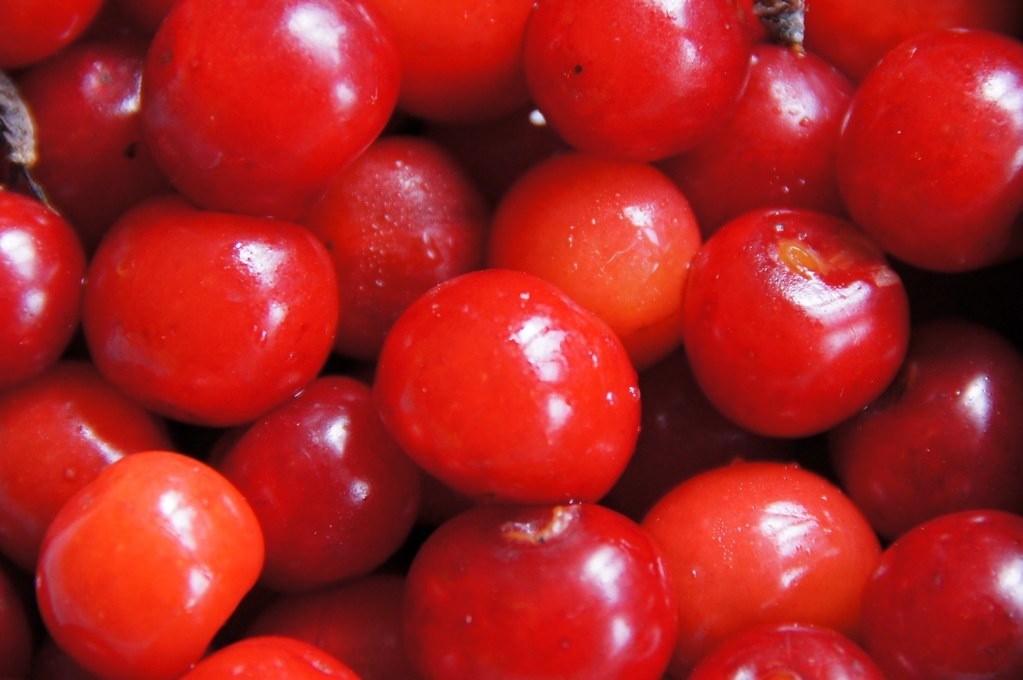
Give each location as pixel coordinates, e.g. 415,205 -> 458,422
0,0 -> 1023,680
